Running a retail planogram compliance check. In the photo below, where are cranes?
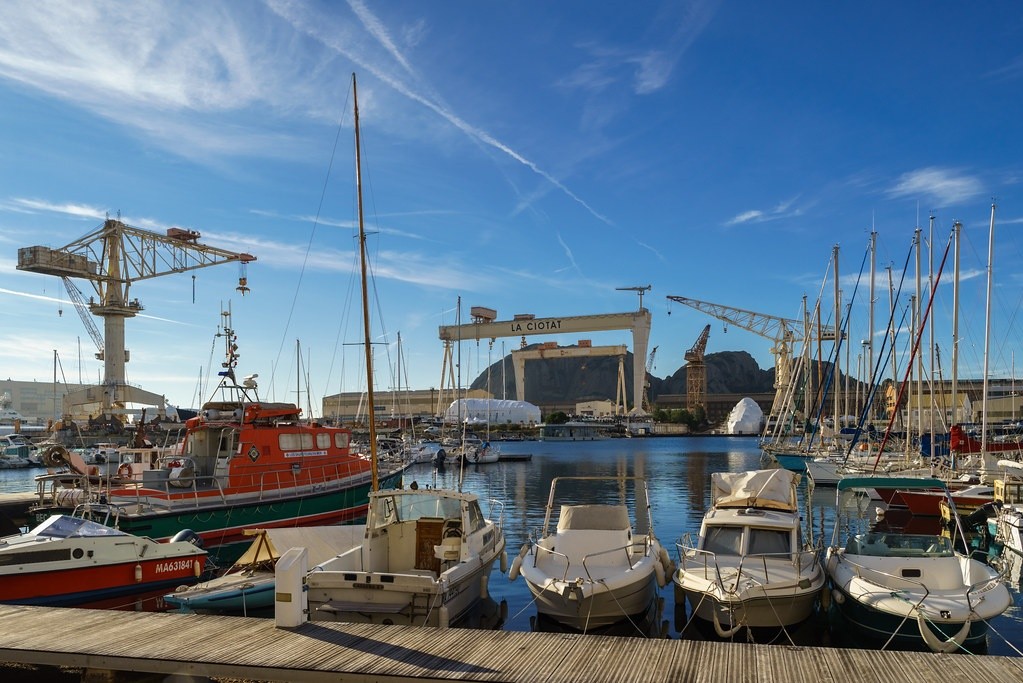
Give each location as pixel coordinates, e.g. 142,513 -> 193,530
16,218 -> 258,432
684,325 -> 711,362
665,295 -> 847,387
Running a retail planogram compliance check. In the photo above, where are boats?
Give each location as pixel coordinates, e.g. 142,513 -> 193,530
825,475 -> 1011,646
93,442 -> 130,462
117,446 -> 169,482
0,434 -> 33,469
26,300 -> 416,567
307,469 -> 506,628
673,469 -> 825,630
0,501 -> 207,608
520,475 -> 662,630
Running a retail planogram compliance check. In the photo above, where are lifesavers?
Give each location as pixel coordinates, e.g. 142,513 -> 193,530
118,463 -> 132,480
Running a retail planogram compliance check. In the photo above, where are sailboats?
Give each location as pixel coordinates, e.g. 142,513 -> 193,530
272,295 -> 500,462
163,72 -> 410,615
758,191 -> 1023,556
28,350 -> 89,464
